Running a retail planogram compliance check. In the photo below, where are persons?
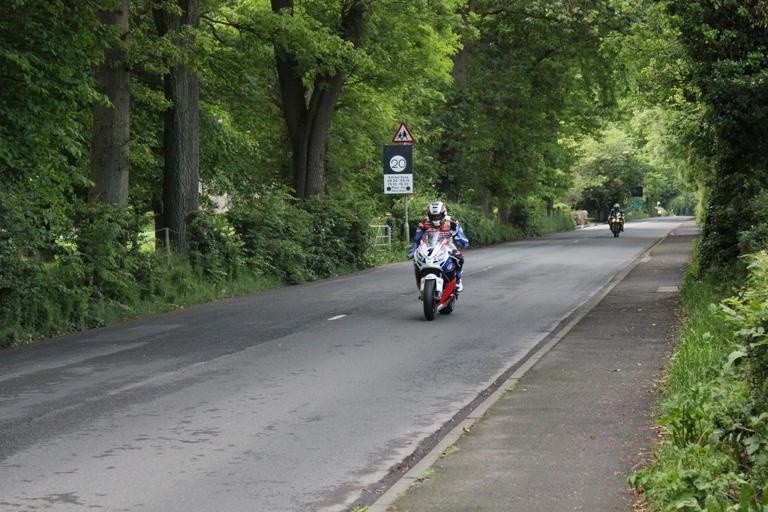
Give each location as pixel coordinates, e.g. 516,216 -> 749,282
406,201 -> 469,300
608,202 -> 624,232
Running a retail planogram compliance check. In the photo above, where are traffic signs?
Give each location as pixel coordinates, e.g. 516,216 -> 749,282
383,145 -> 412,173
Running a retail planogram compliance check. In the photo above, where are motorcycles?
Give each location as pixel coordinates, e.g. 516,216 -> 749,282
407,219 -> 464,320
607,211 -> 624,237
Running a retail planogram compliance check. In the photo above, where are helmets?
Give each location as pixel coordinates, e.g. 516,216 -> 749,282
428,201 -> 447,227
614,203 -> 620,208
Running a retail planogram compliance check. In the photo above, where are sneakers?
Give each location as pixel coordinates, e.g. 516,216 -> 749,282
456,278 -> 462,292
